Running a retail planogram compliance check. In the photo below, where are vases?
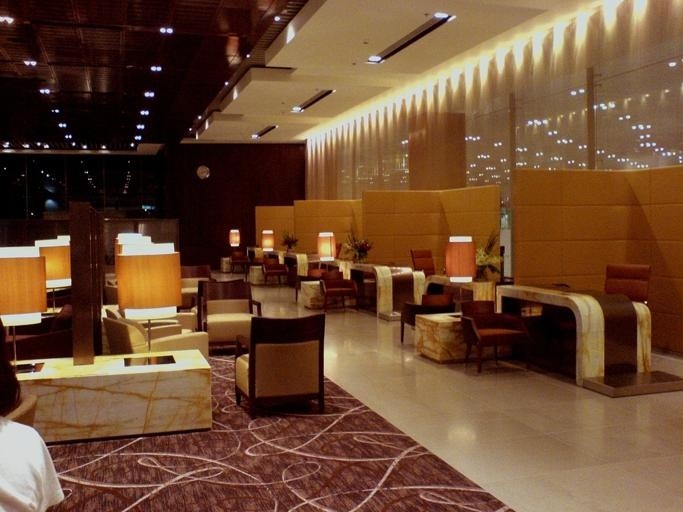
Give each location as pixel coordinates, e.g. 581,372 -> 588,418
355,252 -> 367,264
286,247 -> 295,255
471,268 -> 486,281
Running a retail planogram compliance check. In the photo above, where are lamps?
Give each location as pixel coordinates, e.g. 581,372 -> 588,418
444,236 -> 475,284
115,242 -> 184,367
34,239 -> 73,309
229,229 -> 240,247
0,245 -> 48,377
316,231 -> 336,261
261,229 -> 273,252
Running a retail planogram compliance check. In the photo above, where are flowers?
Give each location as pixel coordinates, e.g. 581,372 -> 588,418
279,231 -> 298,248
474,231 -> 502,276
344,232 -> 373,252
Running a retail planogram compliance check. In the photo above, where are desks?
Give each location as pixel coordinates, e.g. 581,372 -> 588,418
8,348 -> 212,445
421,274 -> 495,302
496,280 -> 651,387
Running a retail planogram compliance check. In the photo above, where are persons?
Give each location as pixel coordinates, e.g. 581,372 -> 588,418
0,317 -> 64,512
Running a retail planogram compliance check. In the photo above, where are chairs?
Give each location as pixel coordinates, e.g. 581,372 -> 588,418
459,300 -> 530,373
196,278 -> 261,353
262,258 -> 288,286
410,248 -> 435,277
400,294 -> 455,343
102,315 -> 209,364
603,262 -> 649,305
228,251 -> 248,274
3,394 -> 37,429
294,268 -> 327,304
319,271 -> 359,314
233,312 -> 326,419
